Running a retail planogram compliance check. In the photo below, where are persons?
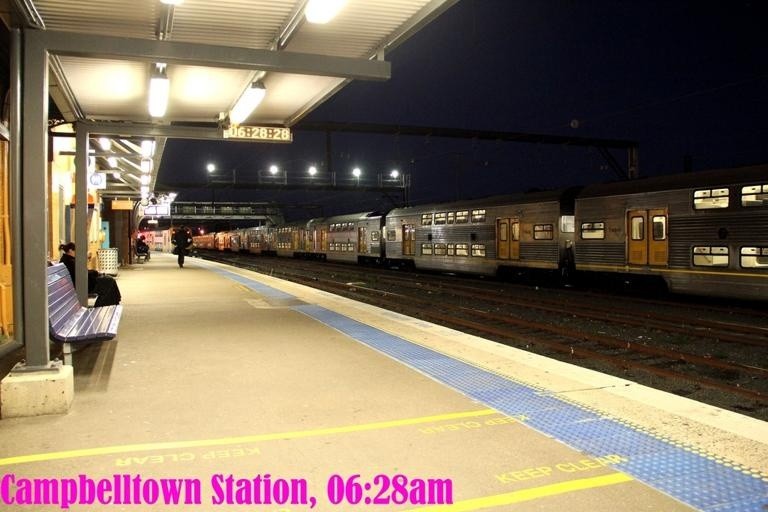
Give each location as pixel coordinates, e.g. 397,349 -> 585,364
136,235 -> 151,262
58,242 -> 121,308
173,225 -> 192,269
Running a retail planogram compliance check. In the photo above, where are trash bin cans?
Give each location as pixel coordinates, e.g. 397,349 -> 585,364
96,248 -> 119,277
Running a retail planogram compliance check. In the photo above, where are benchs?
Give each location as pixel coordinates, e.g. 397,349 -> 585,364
133,239 -> 148,264
45,260 -> 124,366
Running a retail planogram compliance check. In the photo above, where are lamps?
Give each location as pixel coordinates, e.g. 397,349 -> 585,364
146,64 -> 171,119
96,137 -> 154,206
216,71 -> 267,131
